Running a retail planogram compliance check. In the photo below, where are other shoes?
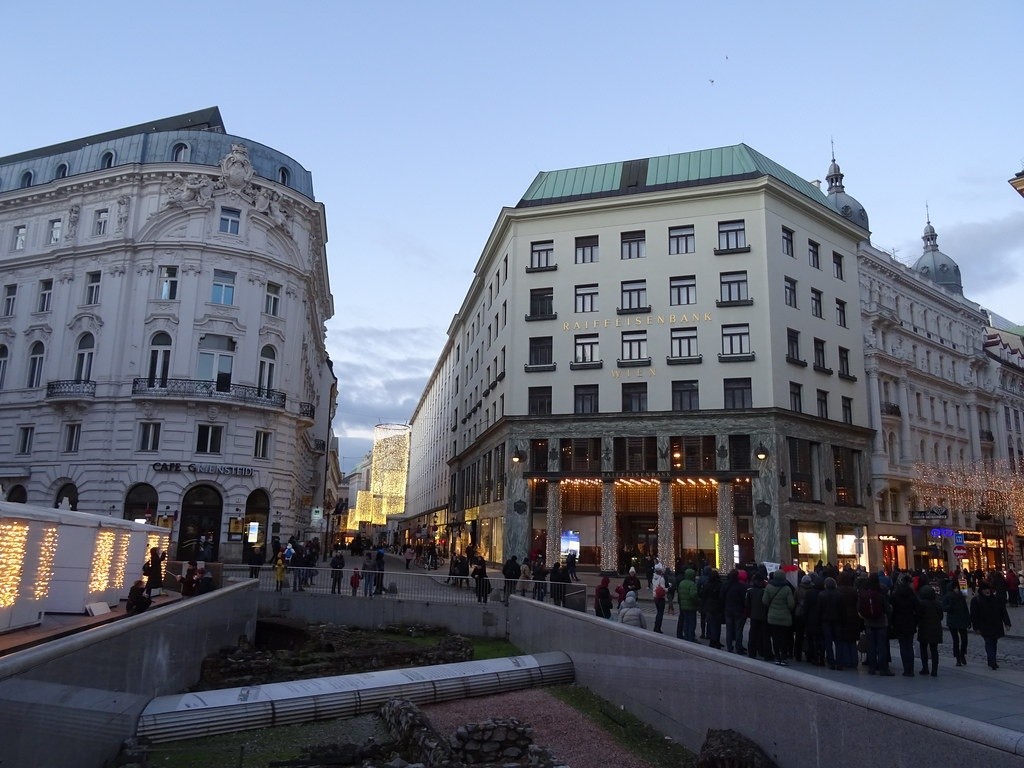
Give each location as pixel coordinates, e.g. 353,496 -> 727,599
919,669 -> 929,674
828,663 -> 835,669
775,659 -> 787,665
654,628 -> 664,633
837,664 -> 843,669
691,638 -> 701,643
709,640 -> 725,649
728,645 -> 735,653
699,632 -> 710,638
862,660 -> 869,664
988,661 -> 999,669
880,668 -> 895,675
737,646 -> 748,654
931,672 -> 937,676
902,671 -> 915,676
956,653 -> 967,665
868,668 -> 875,674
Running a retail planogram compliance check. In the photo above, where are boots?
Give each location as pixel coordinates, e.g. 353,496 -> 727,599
811,655 -> 825,665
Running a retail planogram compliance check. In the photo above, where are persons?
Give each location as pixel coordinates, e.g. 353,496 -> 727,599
126,547 -> 214,619
248,533 -> 395,598
594,547 -> 1024,677
396,542 -> 443,571
445,550 -> 580,606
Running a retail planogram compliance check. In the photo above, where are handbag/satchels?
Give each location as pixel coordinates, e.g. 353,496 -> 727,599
142,554 -> 156,576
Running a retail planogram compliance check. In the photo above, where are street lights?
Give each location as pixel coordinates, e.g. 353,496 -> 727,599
433,513 -> 439,552
109,505 -> 115,516
323,511 -> 331,562
976,489 -> 1009,573
330,518 -> 338,557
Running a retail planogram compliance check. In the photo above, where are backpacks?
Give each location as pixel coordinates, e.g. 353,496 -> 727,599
653,576 -> 665,598
857,589 -> 887,620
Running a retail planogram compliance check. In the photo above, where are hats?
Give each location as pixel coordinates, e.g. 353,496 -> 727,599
629,566 -> 636,573
524,557 -> 529,561
979,581 -> 991,588
774,570 -> 786,579
801,575 -> 812,583
476,559 -> 487,565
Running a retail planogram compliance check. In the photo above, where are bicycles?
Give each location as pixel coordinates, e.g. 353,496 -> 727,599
414,550 -> 444,569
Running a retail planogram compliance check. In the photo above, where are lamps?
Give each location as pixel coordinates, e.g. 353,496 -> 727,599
162,506 -> 170,520
512,445 -> 527,463
276,511 -> 282,522
754,441 -> 769,460
236,508 -> 242,520
108,505 -> 115,516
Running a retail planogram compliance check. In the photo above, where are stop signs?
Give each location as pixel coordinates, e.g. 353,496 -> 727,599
144,510 -> 152,520
953,545 -> 967,558
